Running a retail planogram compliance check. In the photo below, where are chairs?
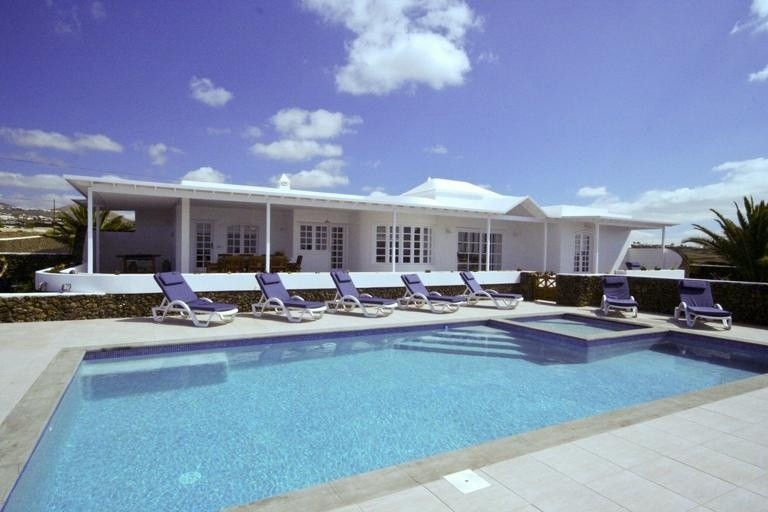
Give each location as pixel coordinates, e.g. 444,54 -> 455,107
397,273 -> 465,314
249,272 -> 328,323
207,252 -> 303,272
459,270 -> 523,310
598,273 -> 737,331
324,268 -> 398,319
154,270 -> 238,326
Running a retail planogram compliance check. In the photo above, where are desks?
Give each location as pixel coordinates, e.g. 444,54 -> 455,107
115,254 -> 161,273
